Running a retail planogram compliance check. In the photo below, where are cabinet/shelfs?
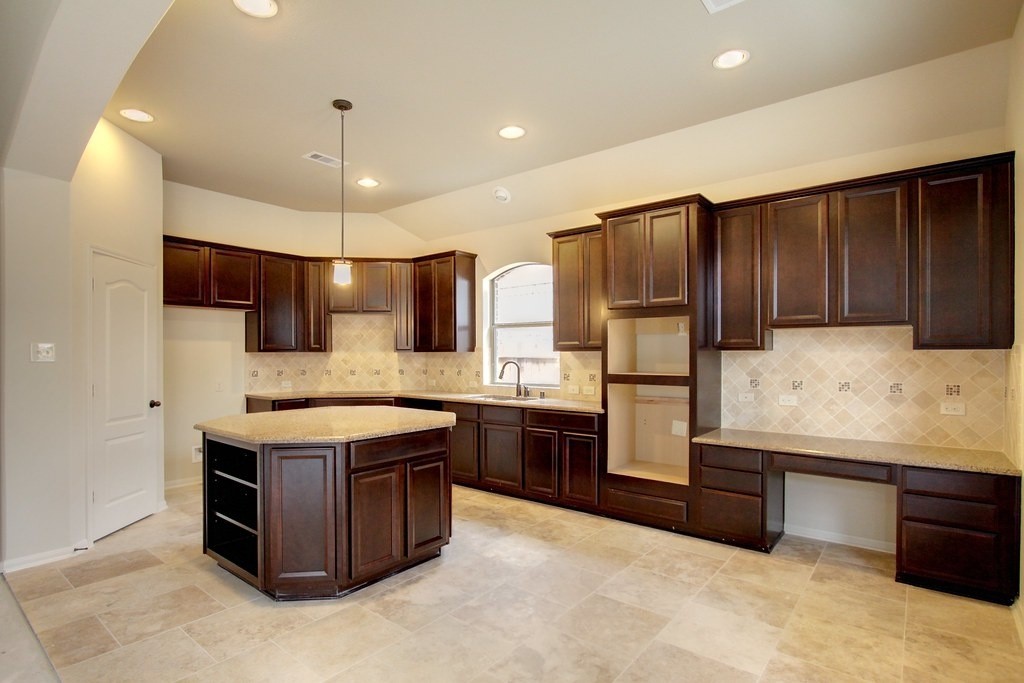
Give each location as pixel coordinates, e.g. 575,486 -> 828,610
163,150 -> 1023,609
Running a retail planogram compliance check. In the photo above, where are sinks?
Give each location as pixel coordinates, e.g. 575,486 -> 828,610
464,394 -> 538,401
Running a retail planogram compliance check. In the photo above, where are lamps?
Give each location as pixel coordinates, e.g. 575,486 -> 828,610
331,99 -> 354,285
493,185 -> 511,203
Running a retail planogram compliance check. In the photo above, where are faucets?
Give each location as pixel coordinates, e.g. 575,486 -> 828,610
499,360 -> 529,397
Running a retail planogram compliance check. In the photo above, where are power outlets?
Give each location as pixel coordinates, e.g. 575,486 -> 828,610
738,393 -> 754,402
672,420 -> 688,436
779,394 -> 797,406
193,446 -> 202,462
940,402 -> 965,415
677,322 -> 687,335
